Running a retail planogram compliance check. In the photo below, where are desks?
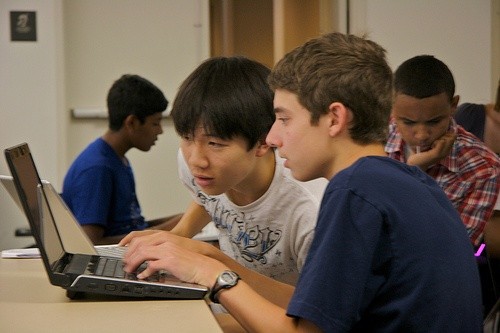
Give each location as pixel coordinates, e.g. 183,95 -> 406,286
0,257 -> 226,333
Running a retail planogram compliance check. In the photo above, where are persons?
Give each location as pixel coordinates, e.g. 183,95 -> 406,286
119,56 -> 321,333
380,56 -> 500,248
122,32 -> 485,333
62,74 -> 186,246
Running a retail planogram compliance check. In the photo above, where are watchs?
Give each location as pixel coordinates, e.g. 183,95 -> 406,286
210,269 -> 241,303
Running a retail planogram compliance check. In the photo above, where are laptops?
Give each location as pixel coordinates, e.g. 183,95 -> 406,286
4,143 -> 208,301
0,175 -> 130,259
